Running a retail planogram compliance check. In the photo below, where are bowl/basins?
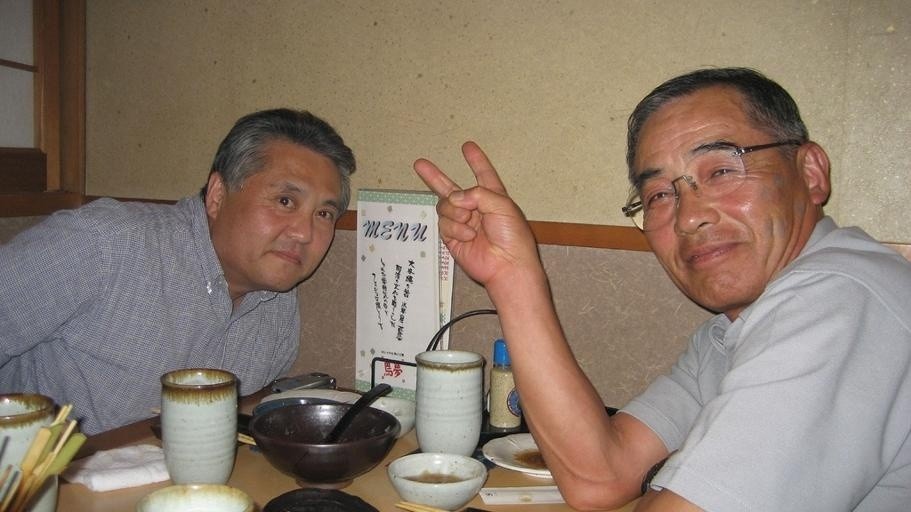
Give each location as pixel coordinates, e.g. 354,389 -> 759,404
387,453 -> 490,508
252,388 -> 415,491
137,485 -> 253,511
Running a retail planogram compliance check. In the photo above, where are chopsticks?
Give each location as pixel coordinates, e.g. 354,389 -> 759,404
151,407 -> 257,447
394,499 -> 451,512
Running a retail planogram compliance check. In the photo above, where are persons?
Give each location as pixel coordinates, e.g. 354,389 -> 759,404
0,105 -> 358,441
413,66 -> 911,512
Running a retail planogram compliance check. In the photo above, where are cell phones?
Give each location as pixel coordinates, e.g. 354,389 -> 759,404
271,372 -> 334,393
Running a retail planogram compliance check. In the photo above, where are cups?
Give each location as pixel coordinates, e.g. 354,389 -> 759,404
413,351 -> 484,460
1,394 -> 61,511
160,367 -> 240,485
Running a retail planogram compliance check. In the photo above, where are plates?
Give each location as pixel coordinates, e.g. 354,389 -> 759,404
481,432 -> 554,479
260,490 -> 380,512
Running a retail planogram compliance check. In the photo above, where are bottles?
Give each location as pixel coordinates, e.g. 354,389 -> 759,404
490,338 -> 522,429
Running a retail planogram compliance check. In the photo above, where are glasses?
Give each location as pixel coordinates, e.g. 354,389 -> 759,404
622,140 -> 802,231
